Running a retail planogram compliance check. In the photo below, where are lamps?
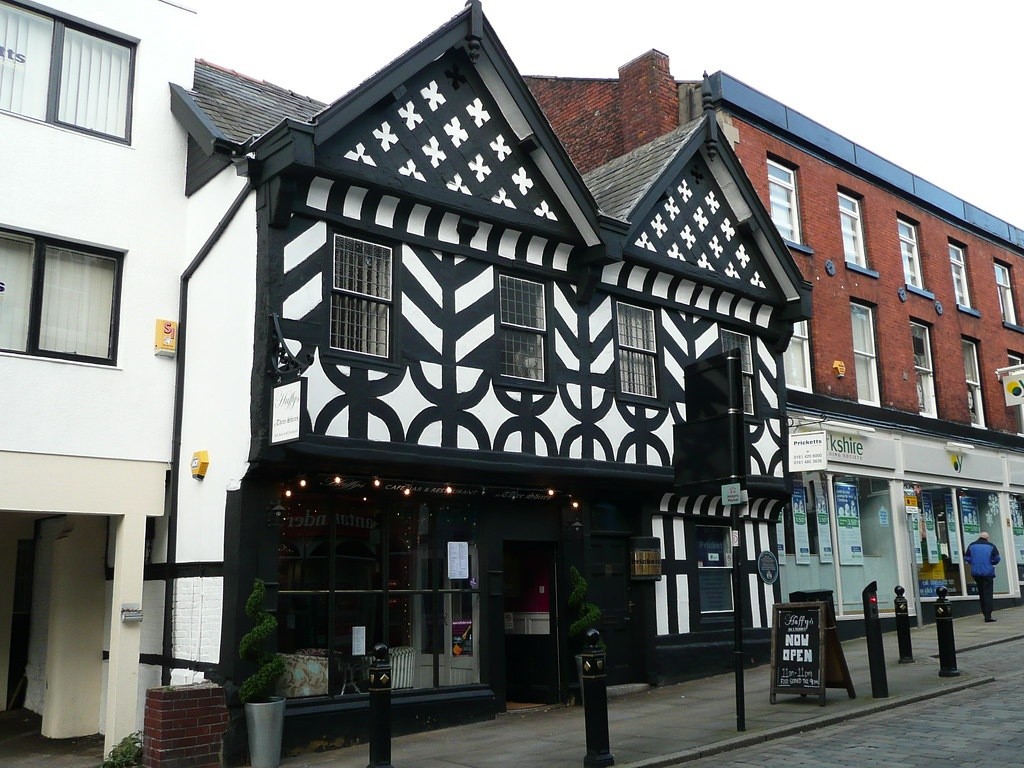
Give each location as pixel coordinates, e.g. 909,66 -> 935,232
800,416 -> 876,437
946,443 -> 975,453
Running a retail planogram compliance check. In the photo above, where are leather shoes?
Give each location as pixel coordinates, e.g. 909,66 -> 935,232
984,618 -> 997,622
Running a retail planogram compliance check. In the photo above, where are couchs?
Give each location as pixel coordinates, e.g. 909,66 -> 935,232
274,649 -> 344,697
359,645 -> 417,691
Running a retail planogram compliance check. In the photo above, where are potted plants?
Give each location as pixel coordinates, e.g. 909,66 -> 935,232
569,565 -> 607,691
238,580 -> 287,768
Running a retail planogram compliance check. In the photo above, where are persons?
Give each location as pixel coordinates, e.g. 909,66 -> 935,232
963,532 -> 1000,622
